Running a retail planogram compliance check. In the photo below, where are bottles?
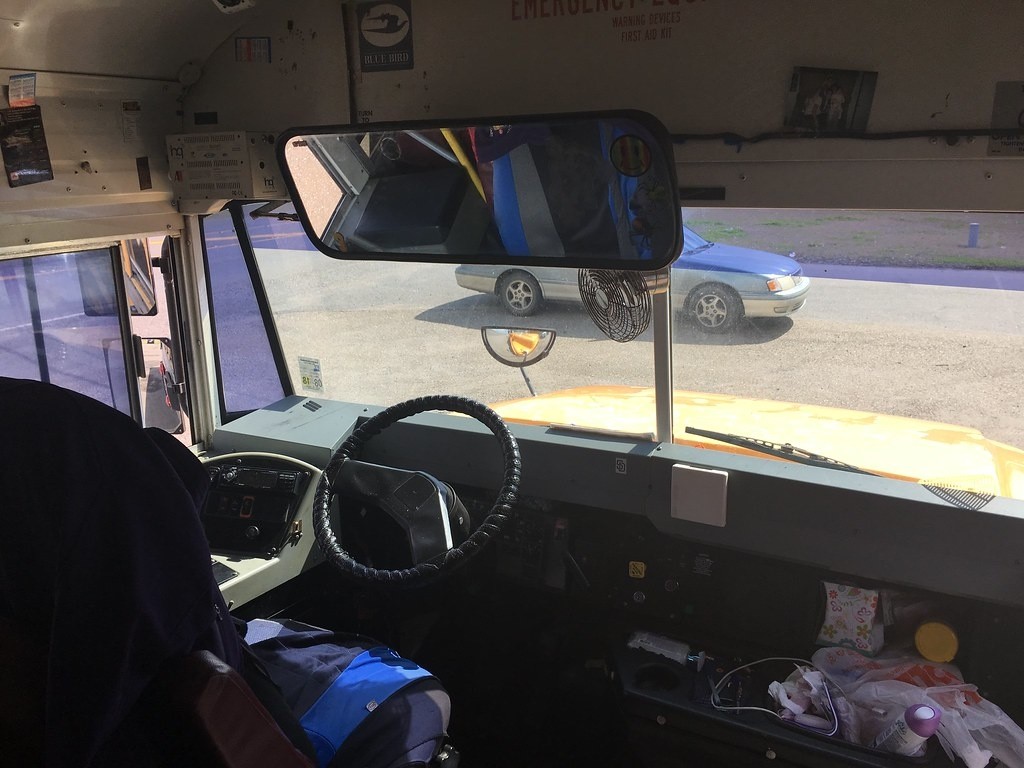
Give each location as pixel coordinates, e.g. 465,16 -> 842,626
867,703 -> 940,756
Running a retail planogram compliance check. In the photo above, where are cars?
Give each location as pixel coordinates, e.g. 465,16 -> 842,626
455,225 -> 811,334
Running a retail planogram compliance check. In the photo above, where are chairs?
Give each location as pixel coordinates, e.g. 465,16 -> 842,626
495,118 -> 667,260
1,377 -> 459,768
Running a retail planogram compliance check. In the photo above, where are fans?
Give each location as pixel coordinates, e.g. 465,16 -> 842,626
578,268 -> 668,343
